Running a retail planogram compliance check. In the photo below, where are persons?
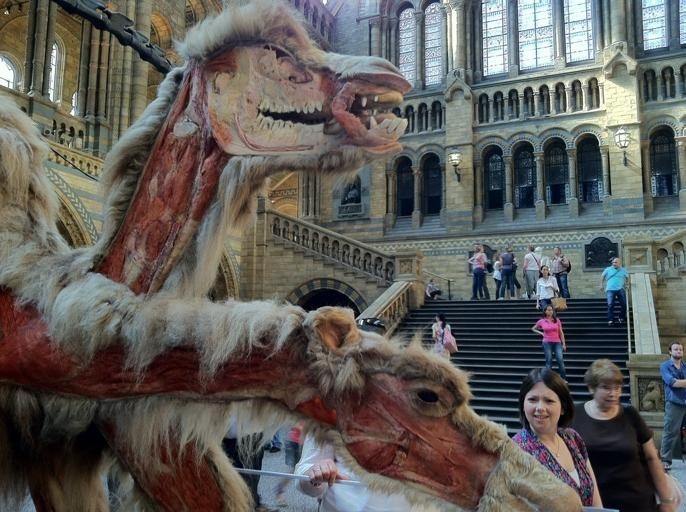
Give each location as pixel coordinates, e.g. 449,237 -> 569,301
272,417 -> 308,507
531,304 -> 569,384
535,265 -> 559,317
563,358 -> 682,512
221,409 -> 281,511
467,244 -> 571,300
293,428 -> 376,512
425,278 -> 447,300
264,428 -> 282,453
600,257 -> 630,327
658,341 -> 686,471
510,368 -> 605,508
431,311 -> 452,362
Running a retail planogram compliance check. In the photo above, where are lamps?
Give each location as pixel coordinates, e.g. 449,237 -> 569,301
613,125 -> 631,167
447,145 -> 464,183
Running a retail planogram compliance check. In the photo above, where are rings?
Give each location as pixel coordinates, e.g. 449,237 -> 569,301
313,468 -> 320,472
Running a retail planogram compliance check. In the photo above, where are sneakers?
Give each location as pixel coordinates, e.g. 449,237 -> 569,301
619,319 -> 624,323
471,297 -> 505,300
663,462 -> 672,470
270,447 -> 280,453
608,321 -> 613,323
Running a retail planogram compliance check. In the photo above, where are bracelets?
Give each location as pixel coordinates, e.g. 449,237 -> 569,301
660,496 -> 676,505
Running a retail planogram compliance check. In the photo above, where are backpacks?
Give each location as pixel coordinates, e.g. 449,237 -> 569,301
553,255 -> 571,273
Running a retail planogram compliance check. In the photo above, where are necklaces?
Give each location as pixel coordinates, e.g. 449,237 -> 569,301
537,432 -> 561,458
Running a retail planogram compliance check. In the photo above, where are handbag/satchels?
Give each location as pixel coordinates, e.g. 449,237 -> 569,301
653,472 -> 683,510
551,292 -> 568,312
443,328 -> 458,354
